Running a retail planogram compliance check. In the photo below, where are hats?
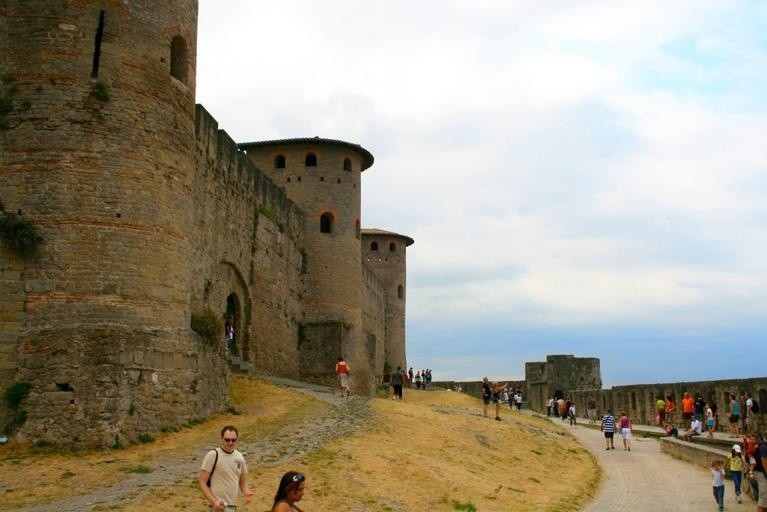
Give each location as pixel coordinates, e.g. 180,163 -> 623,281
732,444 -> 742,453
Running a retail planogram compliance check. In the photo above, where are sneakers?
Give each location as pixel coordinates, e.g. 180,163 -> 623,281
495,416 -> 502,422
734,494 -> 743,504
718,505 -> 724,512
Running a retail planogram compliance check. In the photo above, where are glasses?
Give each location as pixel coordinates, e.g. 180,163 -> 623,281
285,471 -> 305,486
223,437 -> 237,443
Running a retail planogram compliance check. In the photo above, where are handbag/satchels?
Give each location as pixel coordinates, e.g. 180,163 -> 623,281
207,448 -> 219,488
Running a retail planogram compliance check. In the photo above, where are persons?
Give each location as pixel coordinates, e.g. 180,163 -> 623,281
483,376 -> 767,512
382,365 -> 433,400
270,470 -> 305,512
333,356 -> 352,397
199,425 -> 253,511
225,314 -> 235,353
